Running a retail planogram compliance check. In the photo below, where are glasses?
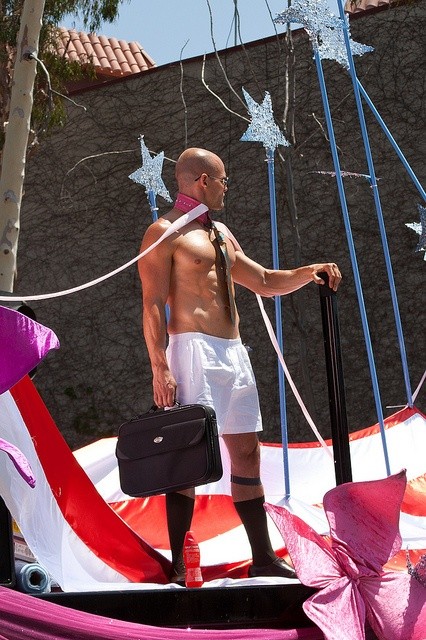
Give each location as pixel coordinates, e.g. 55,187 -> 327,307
194,174 -> 228,187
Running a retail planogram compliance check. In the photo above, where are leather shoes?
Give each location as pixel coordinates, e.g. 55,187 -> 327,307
247,558 -> 297,578
171,561 -> 186,587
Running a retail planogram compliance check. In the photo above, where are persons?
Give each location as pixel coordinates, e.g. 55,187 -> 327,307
137,147 -> 342,588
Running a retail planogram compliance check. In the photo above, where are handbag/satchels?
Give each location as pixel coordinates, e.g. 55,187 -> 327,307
115,401 -> 223,497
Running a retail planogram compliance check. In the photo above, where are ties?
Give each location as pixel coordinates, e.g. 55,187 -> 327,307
205,214 -> 236,327
